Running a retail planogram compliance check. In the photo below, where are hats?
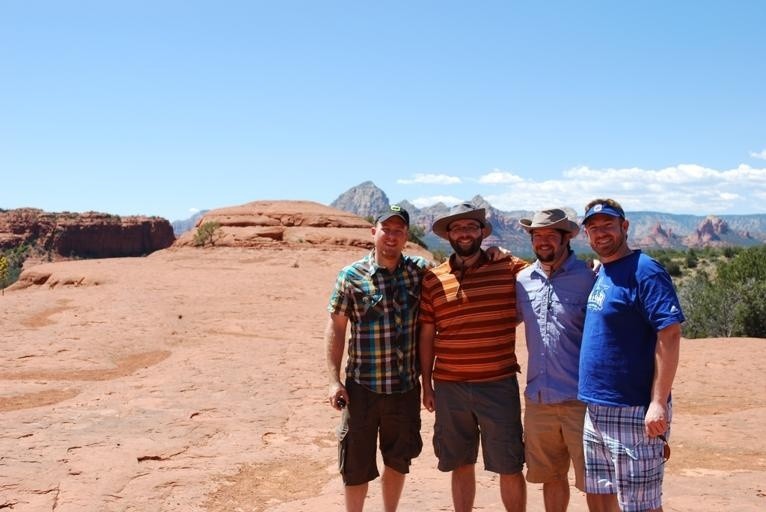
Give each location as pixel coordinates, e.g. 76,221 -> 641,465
432,203 -> 493,241
519,209 -> 580,239
373,206 -> 410,229
581,204 -> 626,225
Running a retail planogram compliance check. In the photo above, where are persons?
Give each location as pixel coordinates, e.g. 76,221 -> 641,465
418,199 -> 602,512
514,208 -> 601,512
576,197 -> 686,512
323,204 -> 513,511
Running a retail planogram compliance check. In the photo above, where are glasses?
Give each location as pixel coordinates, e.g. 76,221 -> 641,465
631,434 -> 671,472
